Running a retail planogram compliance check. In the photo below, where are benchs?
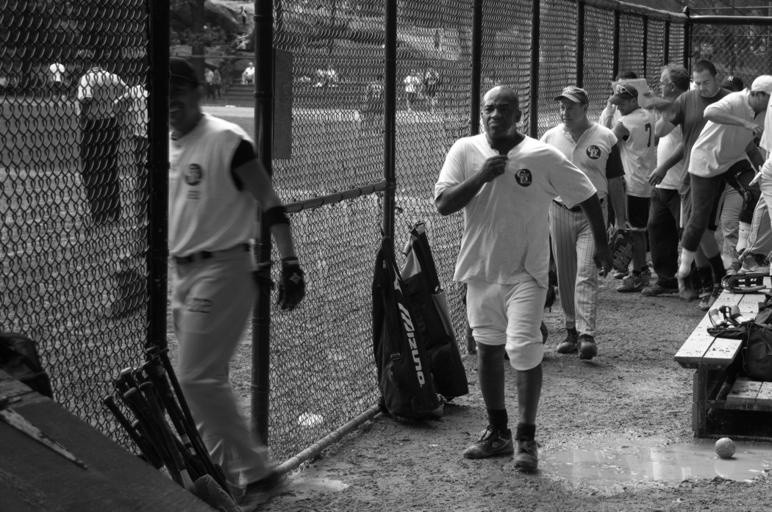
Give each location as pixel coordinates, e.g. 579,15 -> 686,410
673,276 -> 771,441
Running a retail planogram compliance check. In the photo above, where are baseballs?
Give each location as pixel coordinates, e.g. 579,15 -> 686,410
713,438 -> 734,457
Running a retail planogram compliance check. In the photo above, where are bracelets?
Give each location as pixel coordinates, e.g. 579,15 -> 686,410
264,204 -> 291,230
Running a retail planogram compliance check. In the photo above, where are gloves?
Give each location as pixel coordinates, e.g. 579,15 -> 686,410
277,257 -> 305,311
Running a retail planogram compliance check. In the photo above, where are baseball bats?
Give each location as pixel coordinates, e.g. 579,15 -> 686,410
103,345 -> 235,504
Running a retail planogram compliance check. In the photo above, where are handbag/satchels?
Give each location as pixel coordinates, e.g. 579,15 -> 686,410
372,221 -> 468,417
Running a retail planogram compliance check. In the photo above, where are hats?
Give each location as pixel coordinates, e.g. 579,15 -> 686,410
720,76 -> 743,92
751,75 -> 772,95
170,57 -> 198,86
607,86 -> 638,105
553,86 -> 590,104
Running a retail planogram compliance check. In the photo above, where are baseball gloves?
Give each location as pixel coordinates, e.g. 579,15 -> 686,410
608,228 -> 635,273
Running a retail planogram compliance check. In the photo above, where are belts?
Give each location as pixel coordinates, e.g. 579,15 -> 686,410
552,198 -> 604,212
176,243 -> 250,265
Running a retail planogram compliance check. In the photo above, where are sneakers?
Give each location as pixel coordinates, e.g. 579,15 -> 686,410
238,471 -> 292,512
463,425 -> 514,459
557,327 -> 578,354
514,441 -> 538,471
614,270 -> 738,311
578,335 -> 597,359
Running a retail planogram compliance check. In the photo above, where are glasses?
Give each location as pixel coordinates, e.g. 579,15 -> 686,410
616,85 -> 633,97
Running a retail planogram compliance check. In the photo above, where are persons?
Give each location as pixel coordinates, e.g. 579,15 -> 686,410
115,59 -> 310,500
432,84 -> 612,472
112,67 -> 154,228
531,85 -> 633,359
50,58 -> 66,97
66,45 -> 128,231
198,59 -> 256,98
598,57 -> 771,308
298,61 -> 441,124
